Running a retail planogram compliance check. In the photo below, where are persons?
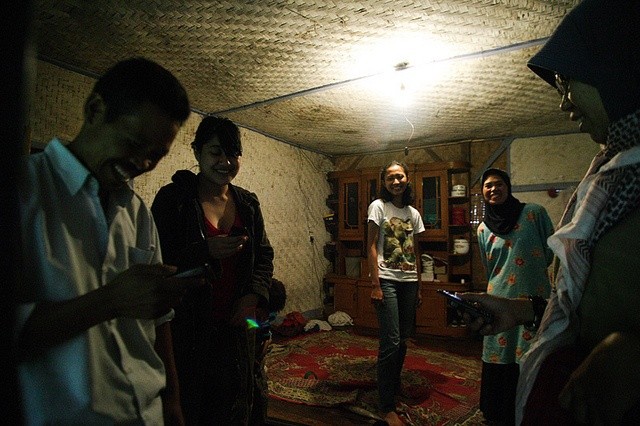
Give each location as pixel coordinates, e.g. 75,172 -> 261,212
455,1 -> 640,426
239,276 -> 284,370
366,159 -> 425,426
477,167 -> 557,426
150,117 -> 273,425
17,57 -> 189,426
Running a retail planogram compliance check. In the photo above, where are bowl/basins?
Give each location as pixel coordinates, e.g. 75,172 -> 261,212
453,239 -> 469,254
450,185 -> 467,191
451,191 -> 466,196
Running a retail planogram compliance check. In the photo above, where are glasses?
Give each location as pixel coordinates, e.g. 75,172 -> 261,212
552,70 -> 569,102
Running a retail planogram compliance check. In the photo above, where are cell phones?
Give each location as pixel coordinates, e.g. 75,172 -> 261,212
171,263 -> 215,281
435,288 -> 494,323
230,223 -> 249,237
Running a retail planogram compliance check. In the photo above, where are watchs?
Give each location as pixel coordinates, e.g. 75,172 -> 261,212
523,295 -> 546,331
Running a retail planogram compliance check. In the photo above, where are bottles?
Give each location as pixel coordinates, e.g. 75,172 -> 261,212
470,193 -> 485,224
452,208 -> 464,224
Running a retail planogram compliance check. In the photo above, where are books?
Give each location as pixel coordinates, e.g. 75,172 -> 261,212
422,252 -> 448,281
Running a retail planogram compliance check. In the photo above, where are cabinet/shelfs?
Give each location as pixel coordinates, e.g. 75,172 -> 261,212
325,160 -> 475,338
